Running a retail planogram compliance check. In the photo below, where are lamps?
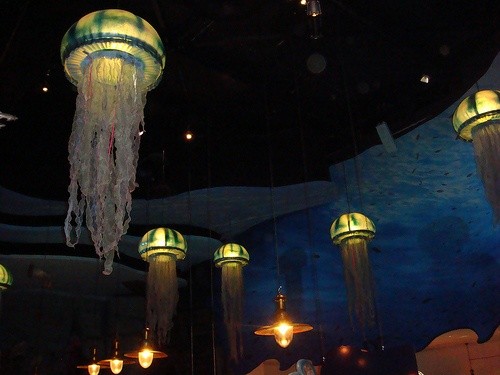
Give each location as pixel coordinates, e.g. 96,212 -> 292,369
1,261 -> 14,291
213,243 -> 250,361
96,337 -> 136,374
420,73 -> 430,83
307,0 -> 322,17
450,87 -> 500,222
76,349 -> 111,375
60,9 -> 168,277
123,327 -> 168,369
138,227 -> 188,344
254,285 -> 314,350
328,212 -> 377,326
